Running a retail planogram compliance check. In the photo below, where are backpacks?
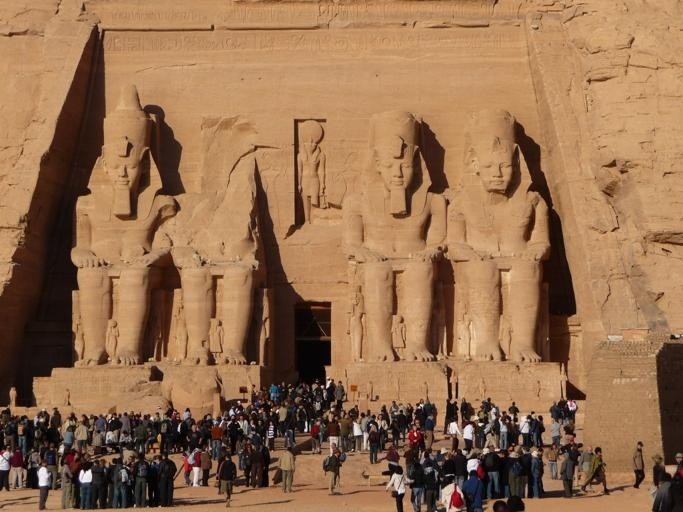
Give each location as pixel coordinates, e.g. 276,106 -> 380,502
323,457 -> 330,472
449,484 -> 463,508
188,452 -> 194,464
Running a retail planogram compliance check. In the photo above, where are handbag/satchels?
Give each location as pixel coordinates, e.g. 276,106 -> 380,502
392,491 -> 398,497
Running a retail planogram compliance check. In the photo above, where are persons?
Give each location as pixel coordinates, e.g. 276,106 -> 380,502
294,118 -> 327,221
341,111 -> 448,362
358,397 -> 609,512
444,108 -> 552,363
631,442 -> 681,512
63,83 -> 175,365
170,185 -> 263,364
233,379 -> 360,495
1,409 -> 269,510
